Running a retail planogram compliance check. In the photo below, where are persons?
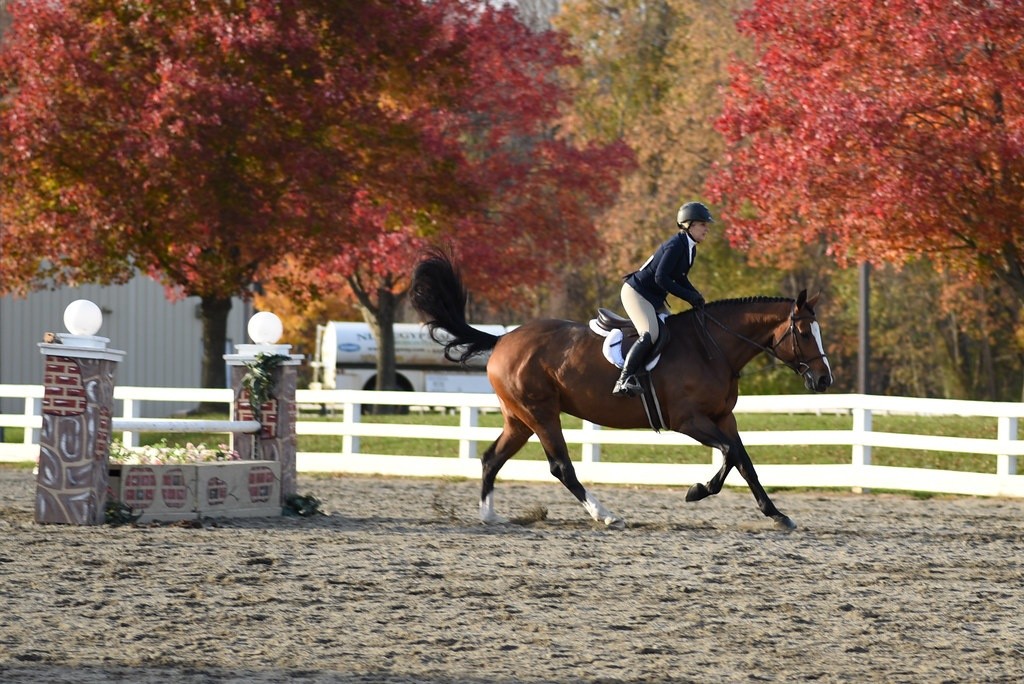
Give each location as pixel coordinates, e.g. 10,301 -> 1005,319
610,202 -> 714,396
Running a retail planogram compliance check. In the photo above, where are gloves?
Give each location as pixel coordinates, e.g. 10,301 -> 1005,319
688,291 -> 705,309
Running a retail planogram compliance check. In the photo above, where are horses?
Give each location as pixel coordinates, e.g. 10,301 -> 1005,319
405,237 -> 835,534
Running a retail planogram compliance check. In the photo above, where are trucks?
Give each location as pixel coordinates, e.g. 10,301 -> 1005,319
322,319 -> 523,415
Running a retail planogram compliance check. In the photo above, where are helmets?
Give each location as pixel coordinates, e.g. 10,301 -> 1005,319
677,202 -> 715,223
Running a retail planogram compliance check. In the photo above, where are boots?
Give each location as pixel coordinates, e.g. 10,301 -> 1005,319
612,332 -> 655,397
